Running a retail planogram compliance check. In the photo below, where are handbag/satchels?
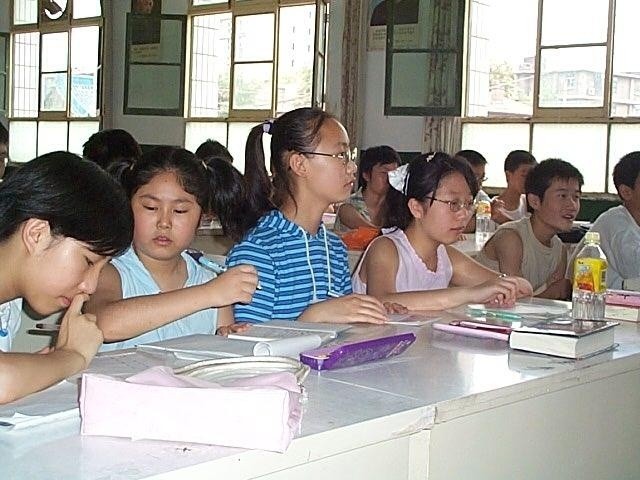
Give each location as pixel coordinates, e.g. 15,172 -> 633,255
80,356 -> 306,453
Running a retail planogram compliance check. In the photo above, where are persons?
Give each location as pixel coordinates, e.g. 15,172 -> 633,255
132,0 -> 160,45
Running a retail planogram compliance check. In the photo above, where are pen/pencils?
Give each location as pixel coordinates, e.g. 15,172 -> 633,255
198,257 -> 264,290
471,310 -> 524,319
36,323 -> 60,330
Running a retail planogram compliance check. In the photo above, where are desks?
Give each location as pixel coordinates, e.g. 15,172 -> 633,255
310,311 -> 640,479
189,220 -> 235,256
322,213 -> 336,229
449,232 -> 496,257
347,250 -> 363,276
0,349 -> 434,479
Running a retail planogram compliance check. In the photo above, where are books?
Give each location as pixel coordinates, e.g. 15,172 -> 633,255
0,379 -> 86,430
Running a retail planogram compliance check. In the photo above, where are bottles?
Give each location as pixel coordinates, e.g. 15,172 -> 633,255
475,196 -> 491,243
571,232 -> 607,319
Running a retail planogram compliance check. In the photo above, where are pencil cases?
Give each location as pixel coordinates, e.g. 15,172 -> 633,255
299,333 -> 417,371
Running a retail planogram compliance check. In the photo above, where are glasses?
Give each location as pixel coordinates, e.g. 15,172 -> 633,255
426,196 -> 475,213
288,147 -> 357,170
